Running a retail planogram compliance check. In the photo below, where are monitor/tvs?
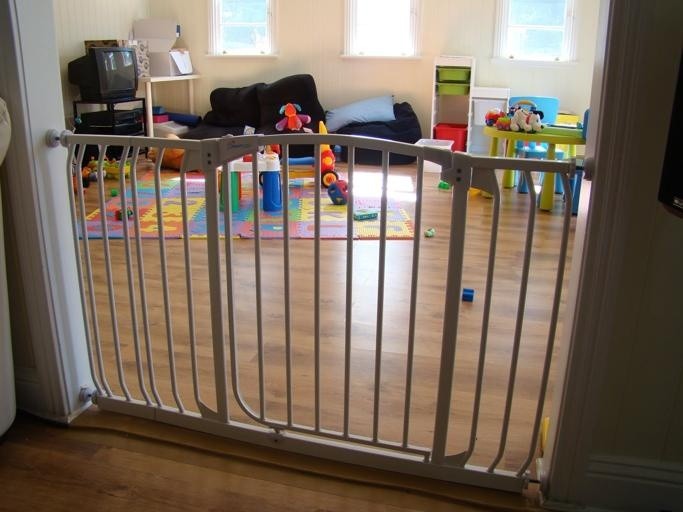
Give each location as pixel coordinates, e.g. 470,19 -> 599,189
68,46 -> 138,101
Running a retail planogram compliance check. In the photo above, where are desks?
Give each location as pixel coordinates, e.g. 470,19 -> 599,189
483,119 -> 584,212
140,73 -> 198,152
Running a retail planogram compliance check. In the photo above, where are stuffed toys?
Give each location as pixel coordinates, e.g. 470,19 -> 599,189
485,100 -> 545,134
319,120 -> 349,205
275,102 -> 311,132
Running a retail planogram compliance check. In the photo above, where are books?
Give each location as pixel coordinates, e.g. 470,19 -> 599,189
353,209 -> 378,220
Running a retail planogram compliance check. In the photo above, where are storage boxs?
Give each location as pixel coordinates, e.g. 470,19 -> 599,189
433,121 -> 468,152
436,66 -> 469,98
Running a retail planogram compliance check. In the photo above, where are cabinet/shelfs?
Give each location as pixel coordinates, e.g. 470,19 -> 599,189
470,86 -> 509,163
71,95 -> 148,161
431,53 -> 473,156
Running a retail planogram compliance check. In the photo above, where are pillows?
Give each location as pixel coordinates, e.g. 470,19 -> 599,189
324,94 -> 397,131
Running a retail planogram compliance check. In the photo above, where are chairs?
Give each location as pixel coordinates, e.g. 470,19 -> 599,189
510,95 -> 591,216
